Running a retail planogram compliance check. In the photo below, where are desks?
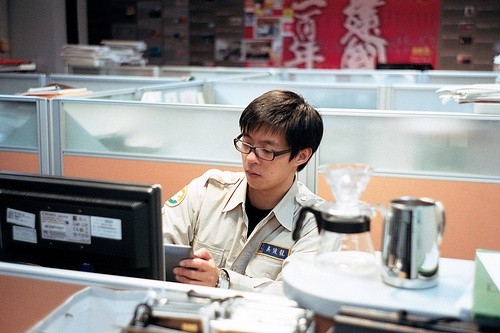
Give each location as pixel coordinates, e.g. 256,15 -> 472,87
282,250 -> 475,320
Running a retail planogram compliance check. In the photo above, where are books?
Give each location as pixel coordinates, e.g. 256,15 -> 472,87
436,83 -> 500,104
19,83 -> 95,97
0,58 -> 36,72
60,40 -> 150,67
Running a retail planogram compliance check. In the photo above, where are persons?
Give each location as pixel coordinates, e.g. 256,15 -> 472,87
161,89 -> 342,295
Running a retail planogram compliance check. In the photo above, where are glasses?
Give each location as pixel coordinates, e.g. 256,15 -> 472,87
234,132 -> 291,160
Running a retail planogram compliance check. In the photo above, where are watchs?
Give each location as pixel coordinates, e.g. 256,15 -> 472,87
215,269 -> 230,289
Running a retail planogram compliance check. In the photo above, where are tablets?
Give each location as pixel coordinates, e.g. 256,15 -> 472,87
162,244 -> 194,283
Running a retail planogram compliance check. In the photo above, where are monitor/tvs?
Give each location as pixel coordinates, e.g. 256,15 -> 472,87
0,170 -> 167,282
